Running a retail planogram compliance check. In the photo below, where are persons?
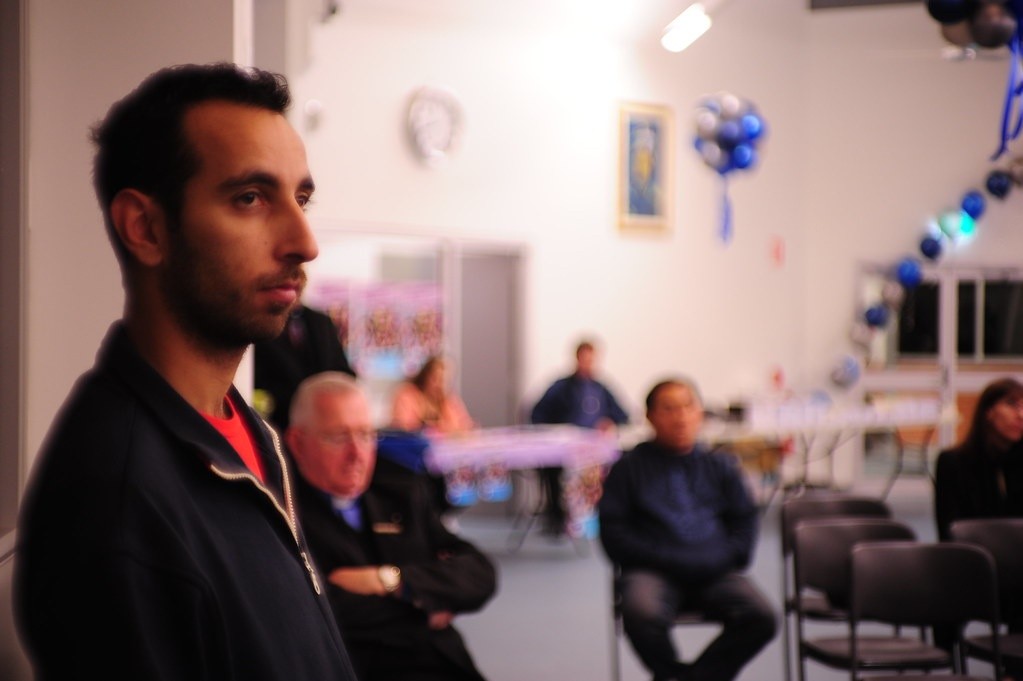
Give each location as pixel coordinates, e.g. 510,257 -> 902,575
937,377 -> 1023,633
11,62 -> 498,681
390,356 -> 471,436
597,381 -> 777,681
531,343 -> 625,534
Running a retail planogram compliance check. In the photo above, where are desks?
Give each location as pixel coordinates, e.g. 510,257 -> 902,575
616,421 -> 788,523
751,399 -> 946,504
425,426 -> 613,557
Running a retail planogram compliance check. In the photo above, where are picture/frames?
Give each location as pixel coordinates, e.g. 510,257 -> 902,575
616,102 -> 678,240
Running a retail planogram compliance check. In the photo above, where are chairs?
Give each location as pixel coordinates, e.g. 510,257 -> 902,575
952,520 -> 1023,681
606,560 -> 722,681
779,497 -> 891,681
849,542 -> 999,681
791,521 -> 918,681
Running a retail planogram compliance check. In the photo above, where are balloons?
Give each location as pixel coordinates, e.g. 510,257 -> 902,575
926,0 -> 1023,51
695,90 -> 763,172
831,157 -> 1023,385
